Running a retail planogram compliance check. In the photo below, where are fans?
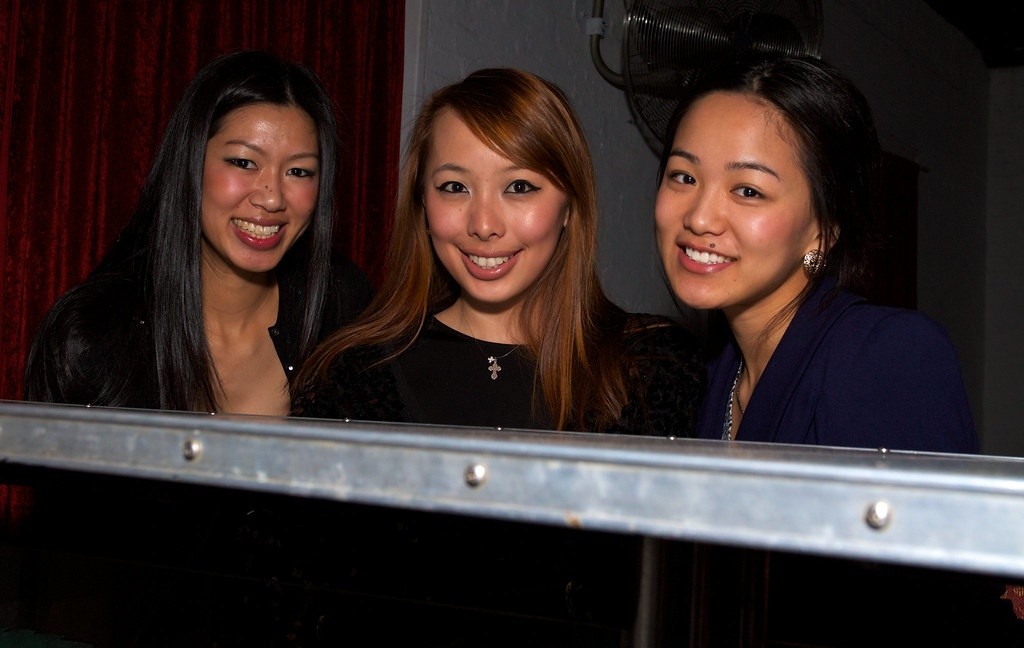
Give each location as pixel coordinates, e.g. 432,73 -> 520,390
589,0 -> 824,148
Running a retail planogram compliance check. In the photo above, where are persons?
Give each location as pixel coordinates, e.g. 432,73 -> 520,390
653,49 -> 1020,648
291,68 -> 715,648
20,48 -> 382,648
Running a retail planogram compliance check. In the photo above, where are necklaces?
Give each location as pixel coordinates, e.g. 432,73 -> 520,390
735,362 -> 747,415
460,293 -> 532,381
